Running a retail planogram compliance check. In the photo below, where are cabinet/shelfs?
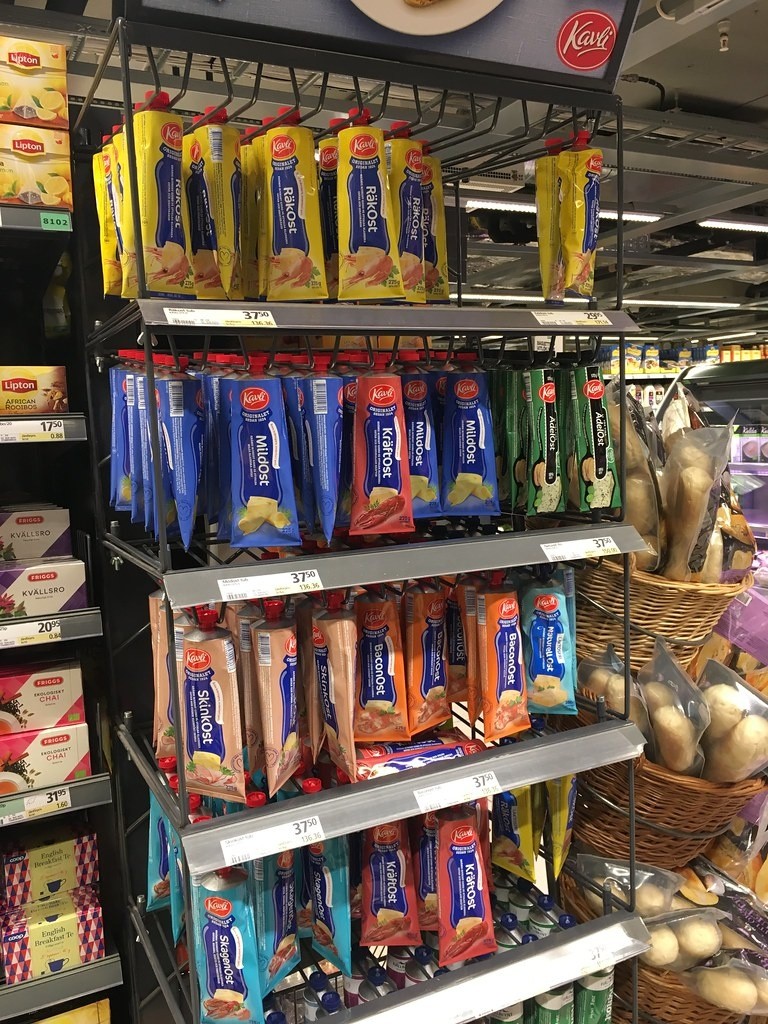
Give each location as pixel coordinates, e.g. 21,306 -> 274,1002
601,368 -> 678,411
0,202 -> 124,1024
727,461 -> 768,601
91,295 -> 654,1024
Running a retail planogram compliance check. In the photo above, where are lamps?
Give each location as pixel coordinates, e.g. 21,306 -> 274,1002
465,199 -> 768,242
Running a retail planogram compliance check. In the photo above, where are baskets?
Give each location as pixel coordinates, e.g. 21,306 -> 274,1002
574,556 -> 755,683
562,871 -> 763,1023
548,684 -> 765,870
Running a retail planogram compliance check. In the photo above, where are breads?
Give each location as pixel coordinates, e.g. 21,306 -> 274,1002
607,404 -> 723,582
588,876 -> 768,1012
589,667 -> 768,784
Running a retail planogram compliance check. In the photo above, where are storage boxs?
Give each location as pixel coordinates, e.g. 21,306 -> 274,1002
556,871 -> 768,1024
575,555 -> 756,674
572,744 -> 768,872
